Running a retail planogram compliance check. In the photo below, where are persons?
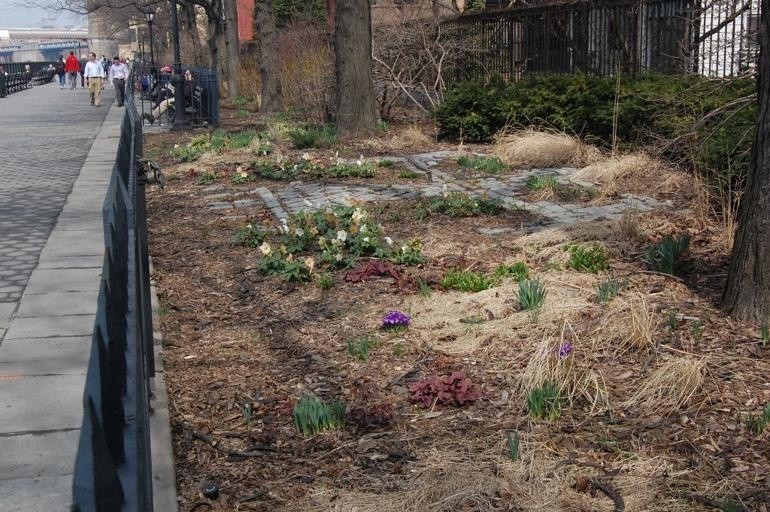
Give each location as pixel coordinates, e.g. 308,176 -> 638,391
0,50 -> 197,124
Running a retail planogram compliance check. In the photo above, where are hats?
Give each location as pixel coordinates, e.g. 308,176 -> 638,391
163,66 -> 172,72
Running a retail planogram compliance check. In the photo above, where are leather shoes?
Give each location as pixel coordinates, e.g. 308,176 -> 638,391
145,112 -> 155,122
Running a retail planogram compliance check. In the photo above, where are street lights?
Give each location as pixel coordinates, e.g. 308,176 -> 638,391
144,7 -> 155,66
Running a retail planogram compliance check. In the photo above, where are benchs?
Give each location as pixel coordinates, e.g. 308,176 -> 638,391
0,72 -> 31,98
33,70 -> 54,86
169,85 -> 204,124
